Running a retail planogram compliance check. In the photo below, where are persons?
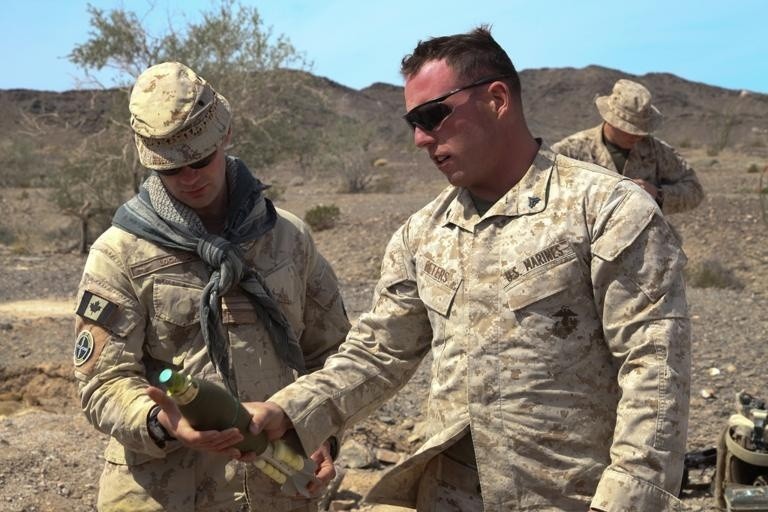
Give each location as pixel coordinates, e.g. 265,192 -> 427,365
549,79 -> 704,214
74,62 -> 351,512
204,24 -> 691,512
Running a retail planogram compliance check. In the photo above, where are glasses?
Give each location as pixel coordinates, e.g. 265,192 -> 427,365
154,150 -> 217,176
401,78 -> 491,133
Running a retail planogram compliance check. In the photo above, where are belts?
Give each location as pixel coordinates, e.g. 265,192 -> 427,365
425,453 -> 483,496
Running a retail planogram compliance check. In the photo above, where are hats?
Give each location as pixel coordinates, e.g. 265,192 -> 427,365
596,78 -> 662,135
129,61 -> 231,171
